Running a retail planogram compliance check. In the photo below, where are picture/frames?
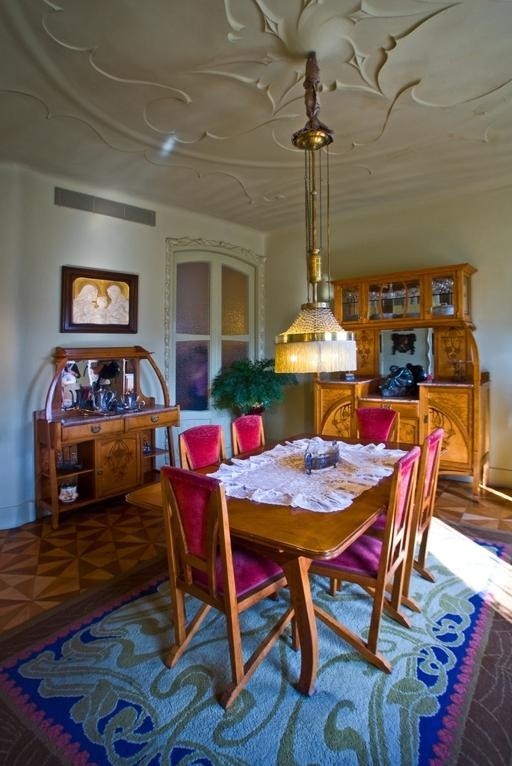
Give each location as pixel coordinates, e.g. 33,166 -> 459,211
61,266 -> 138,334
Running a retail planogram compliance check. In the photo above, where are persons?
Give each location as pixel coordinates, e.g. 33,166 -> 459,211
73,280 -> 129,325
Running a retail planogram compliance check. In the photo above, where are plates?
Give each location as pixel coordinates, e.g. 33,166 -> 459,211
370,312 -> 420,320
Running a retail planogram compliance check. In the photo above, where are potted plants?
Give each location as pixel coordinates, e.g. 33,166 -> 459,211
211,359 -> 298,415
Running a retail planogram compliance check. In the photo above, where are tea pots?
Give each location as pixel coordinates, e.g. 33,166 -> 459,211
93,386 -> 140,413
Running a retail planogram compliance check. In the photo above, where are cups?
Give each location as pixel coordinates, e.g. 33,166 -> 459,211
346,374 -> 355,381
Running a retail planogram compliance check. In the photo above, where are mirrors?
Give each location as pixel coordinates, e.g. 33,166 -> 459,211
60,356 -> 137,411
380,328 -> 434,379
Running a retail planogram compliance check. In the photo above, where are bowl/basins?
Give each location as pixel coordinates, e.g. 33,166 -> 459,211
431,302 -> 454,315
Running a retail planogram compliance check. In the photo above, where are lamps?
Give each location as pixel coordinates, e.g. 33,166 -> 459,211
274,51 -> 357,374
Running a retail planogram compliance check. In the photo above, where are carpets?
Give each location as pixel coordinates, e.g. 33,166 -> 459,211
0,517 -> 512,766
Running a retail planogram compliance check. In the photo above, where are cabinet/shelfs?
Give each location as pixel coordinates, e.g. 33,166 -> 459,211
313,264 -> 490,503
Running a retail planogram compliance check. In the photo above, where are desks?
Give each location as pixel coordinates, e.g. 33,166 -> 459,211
126,433 -> 419,697
35,345 -> 180,529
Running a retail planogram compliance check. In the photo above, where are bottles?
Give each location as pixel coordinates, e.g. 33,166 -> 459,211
432,280 -> 453,293
59,484 -> 79,503
143,440 -> 153,456
55,450 -> 77,473
345,291 -> 357,302
368,283 -> 418,300
